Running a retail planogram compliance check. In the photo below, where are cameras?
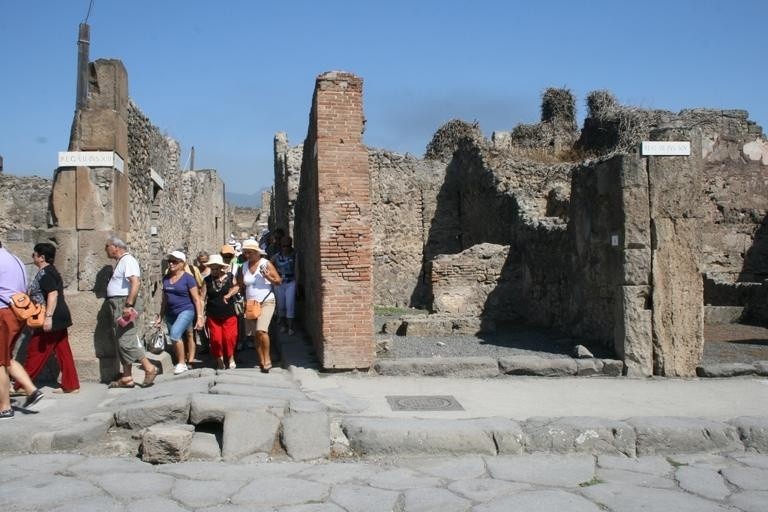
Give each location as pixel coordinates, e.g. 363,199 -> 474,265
259,264 -> 268,274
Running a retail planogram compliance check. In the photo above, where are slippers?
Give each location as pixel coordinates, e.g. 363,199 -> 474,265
261,360 -> 272,371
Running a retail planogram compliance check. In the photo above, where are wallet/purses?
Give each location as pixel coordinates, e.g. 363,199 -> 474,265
116,308 -> 138,329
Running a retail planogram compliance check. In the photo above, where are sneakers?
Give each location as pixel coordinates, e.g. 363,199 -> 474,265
228,359 -> 237,370
218,357 -> 226,370
23,389 -> 44,408
0,408 -> 14,419
279,326 -> 294,335
173,363 -> 188,374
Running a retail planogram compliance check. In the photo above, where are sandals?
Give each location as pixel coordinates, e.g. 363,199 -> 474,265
52,388 -> 80,394
142,364 -> 160,388
9,386 -> 28,397
108,378 -> 135,388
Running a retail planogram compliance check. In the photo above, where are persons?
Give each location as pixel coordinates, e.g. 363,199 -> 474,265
8,243 -> 80,396
104,237 -> 160,388
163,231 -> 299,375
0,243 -> 45,419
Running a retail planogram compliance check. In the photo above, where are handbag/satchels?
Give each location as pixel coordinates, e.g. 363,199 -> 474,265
26,302 -> 46,328
10,293 -> 36,321
143,323 -> 167,354
232,291 -> 245,315
244,299 -> 262,320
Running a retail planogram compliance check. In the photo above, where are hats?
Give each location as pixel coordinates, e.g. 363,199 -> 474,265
203,254 -> 229,269
241,239 -> 268,255
221,245 -> 234,255
165,251 -> 186,264
